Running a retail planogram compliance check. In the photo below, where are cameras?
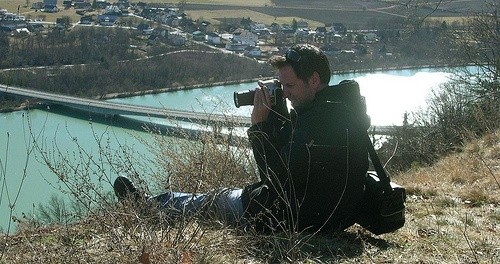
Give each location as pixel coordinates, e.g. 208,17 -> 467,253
234,79 -> 283,109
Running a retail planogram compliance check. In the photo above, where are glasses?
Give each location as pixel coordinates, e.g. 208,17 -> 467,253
285,48 -> 316,74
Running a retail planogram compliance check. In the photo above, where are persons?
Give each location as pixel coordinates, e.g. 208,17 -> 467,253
114,44 -> 371,240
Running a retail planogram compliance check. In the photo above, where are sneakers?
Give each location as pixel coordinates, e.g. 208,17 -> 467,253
113,174 -> 146,212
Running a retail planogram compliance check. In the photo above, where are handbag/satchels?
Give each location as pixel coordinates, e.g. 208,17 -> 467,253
352,167 -> 407,236
